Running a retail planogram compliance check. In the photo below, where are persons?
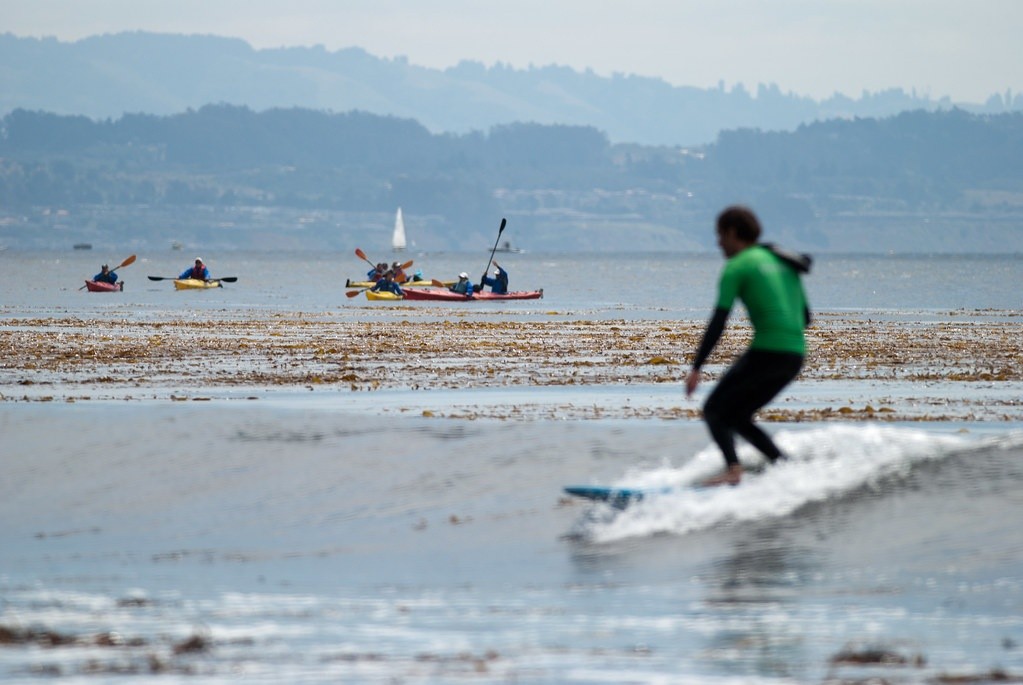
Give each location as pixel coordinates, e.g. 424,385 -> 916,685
177,257 -> 210,280
686,206 -> 810,487
482,261 -> 508,293
370,271 -> 407,296
450,272 -> 473,297
367,262 -> 407,282
93,264 -> 118,284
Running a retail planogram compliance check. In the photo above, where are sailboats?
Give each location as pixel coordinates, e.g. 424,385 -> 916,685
392,206 -> 407,252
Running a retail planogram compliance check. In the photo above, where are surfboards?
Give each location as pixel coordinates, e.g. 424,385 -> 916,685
564,484 -> 736,512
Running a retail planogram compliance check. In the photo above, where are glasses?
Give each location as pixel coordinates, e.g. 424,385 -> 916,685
393,263 -> 403,269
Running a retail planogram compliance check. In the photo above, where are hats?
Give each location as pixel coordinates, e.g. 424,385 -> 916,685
194,257 -> 203,264
382,269 -> 393,275
494,269 -> 501,275
458,272 -> 468,278
102,264 -> 109,269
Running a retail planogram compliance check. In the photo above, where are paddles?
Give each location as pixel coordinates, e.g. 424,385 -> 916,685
79,254 -> 137,291
147,275 -> 238,283
355,247 -> 378,270
345,274 -> 406,299
432,278 -> 479,300
402,260 -> 414,269
481,217 -> 507,289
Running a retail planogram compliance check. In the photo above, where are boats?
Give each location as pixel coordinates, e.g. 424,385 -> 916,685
490,246 -> 521,253
365,289 -> 404,301
85,280 -> 124,291
175,279 -> 221,290
403,287 -> 543,299
345,279 -> 458,285
